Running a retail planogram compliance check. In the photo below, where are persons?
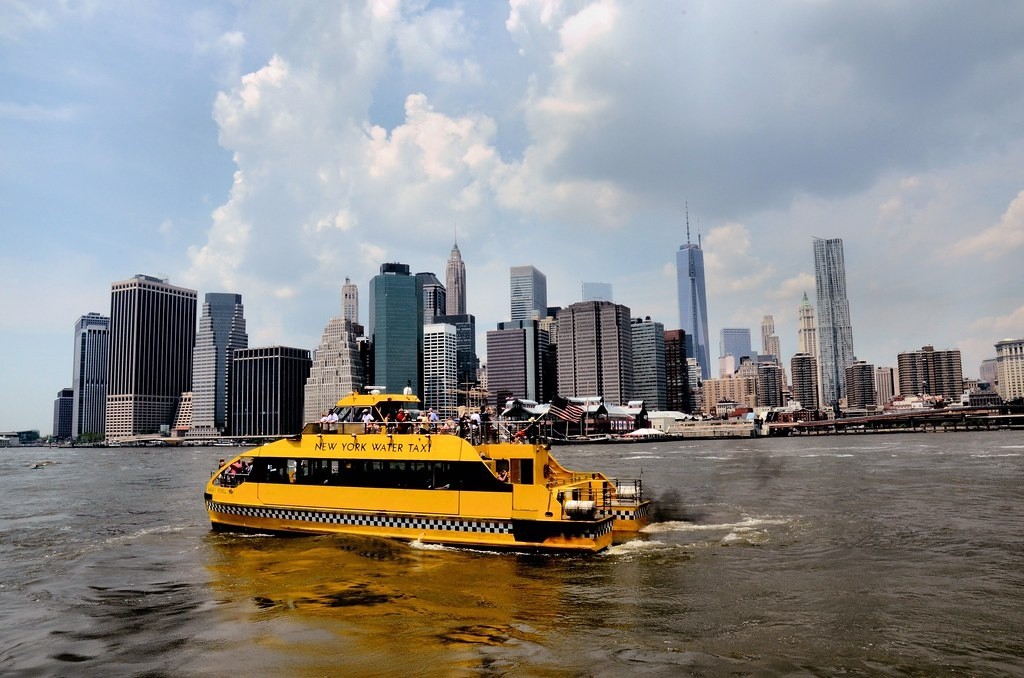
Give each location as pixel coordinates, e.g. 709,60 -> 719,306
316,409 -> 339,431
417,407 -> 437,434
397,409 -> 407,433
528,417 -> 538,444
385,412 -> 391,426
219,458 -> 253,487
445,406 -> 493,445
497,468 -> 508,481
373,420 -> 380,429
293,460 -> 304,477
361,409 -> 374,425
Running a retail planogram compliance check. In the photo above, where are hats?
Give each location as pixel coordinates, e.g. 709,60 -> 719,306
528,417 -> 537,422
362,408 -> 368,413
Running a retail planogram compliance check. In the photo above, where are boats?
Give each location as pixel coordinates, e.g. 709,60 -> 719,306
202,394 -> 617,553
607,477 -> 650,541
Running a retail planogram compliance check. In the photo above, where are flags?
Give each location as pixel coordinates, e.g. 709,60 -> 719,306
549,396 -> 584,424
